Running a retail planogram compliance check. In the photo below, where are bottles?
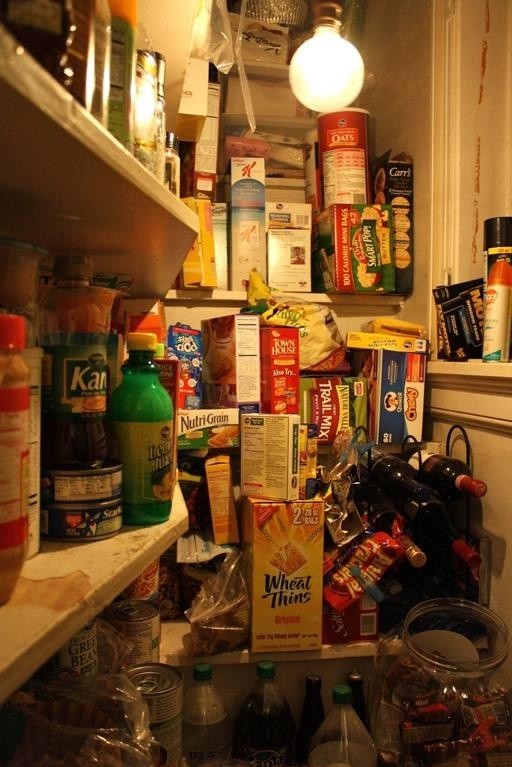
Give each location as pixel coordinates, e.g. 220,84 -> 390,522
106,0 -> 135,155
359,446 -> 428,498
109,332 -> 174,524
164,131 -> 180,199
133,18 -> 155,168
398,448 -> 488,498
291,676 -> 325,767
232,661 -> 297,767
400,496 -> 482,568
308,686 -> 377,767
182,664 -> 232,767
0,313 -> 63,606
349,672 -> 371,738
91,0 -> 111,126
331,470 -> 425,567
48,327 -> 110,471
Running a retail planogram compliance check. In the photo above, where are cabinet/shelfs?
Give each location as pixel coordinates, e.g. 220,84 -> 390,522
164,64 -> 439,457
1,23 -> 200,671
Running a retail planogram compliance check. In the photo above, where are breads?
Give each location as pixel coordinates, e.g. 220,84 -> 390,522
37,693 -> 166,767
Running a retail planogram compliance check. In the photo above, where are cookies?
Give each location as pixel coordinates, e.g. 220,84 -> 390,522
393,232 -> 412,249
392,197 -> 411,213
393,249 -> 411,269
393,214 -> 411,232
272,544 -> 307,575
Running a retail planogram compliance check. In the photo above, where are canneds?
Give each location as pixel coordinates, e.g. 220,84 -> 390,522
124,663 -> 182,767
41,460 -> 123,504
39,502 -> 123,542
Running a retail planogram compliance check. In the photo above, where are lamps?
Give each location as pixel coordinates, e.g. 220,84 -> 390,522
288,0 -> 364,114
